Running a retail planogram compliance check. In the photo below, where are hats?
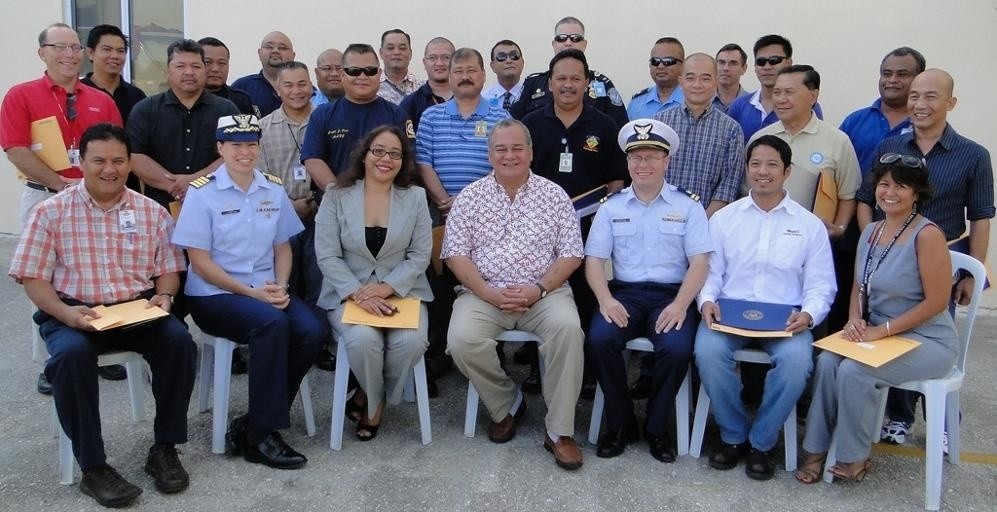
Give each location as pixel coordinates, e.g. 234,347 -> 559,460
215,113 -> 263,142
617,118 -> 680,155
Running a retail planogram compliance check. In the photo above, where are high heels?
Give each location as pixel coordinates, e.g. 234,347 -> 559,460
357,393 -> 386,441
824,457 -> 874,484
795,453 -> 826,485
347,378 -> 367,421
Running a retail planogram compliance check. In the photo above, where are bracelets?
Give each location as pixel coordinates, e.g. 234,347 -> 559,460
888,320 -> 892,338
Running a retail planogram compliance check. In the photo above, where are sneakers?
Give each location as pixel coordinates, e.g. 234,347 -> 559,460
941,424 -> 953,458
879,416 -> 917,444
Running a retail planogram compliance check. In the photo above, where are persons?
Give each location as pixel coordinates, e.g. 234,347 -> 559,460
229,32 -> 319,121
308,49 -> 347,110
439,120 -> 586,471
838,48 -> 927,249
129,40 -> 249,376
171,114 -> 326,470
727,35 -> 823,147
397,38 -> 456,134
252,60 -> 337,371
857,69 -> 995,457
585,119 -> 715,463
7,124 -> 196,508
376,29 -> 428,106
797,154 -> 960,485
313,125 -> 434,441
300,45 -> 439,398
415,48 -> 514,377
508,17 -> 630,131
631,53 -> 745,399
80,24 -> 148,193
0,24 -> 129,394
523,48 -> 625,400
196,38 -> 254,114
627,37 -> 686,120
480,40 -> 525,113
697,136 -> 838,481
710,44 -> 748,118
740,65 -> 863,424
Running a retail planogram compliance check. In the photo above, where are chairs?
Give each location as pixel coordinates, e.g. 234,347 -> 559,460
51,350 -> 153,489
462,329 -> 545,437
330,335 -> 433,450
196,334 -> 316,453
688,347 -> 798,471
587,336 -> 691,456
823,249 -> 985,511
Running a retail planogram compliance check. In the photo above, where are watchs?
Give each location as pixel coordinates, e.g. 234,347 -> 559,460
159,292 -> 174,300
534,283 -> 548,299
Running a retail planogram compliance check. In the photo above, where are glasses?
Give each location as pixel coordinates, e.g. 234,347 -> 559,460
880,152 -> 926,169
492,50 -> 522,61
64,94 -> 77,119
367,147 -> 403,160
553,33 -> 584,43
754,55 -> 789,66
648,56 -> 683,66
41,41 -> 86,54
342,65 -> 380,76
427,54 -> 452,61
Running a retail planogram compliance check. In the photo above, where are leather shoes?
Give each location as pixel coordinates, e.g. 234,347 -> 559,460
34,368 -> 54,395
595,420 -> 640,459
709,440 -> 750,470
144,443 -> 191,494
226,411 -> 247,452
320,349 -> 336,369
645,428 -> 678,464
583,377 -> 597,402
80,464 -> 144,507
747,445 -> 777,481
487,391 -> 527,443
237,430 -> 307,469
101,364 -> 128,381
543,426 -> 584,471
522,362 -> 540,391
232,351 -> 244,374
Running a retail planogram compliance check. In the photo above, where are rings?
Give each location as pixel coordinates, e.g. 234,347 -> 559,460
177,195 -> 182,199
